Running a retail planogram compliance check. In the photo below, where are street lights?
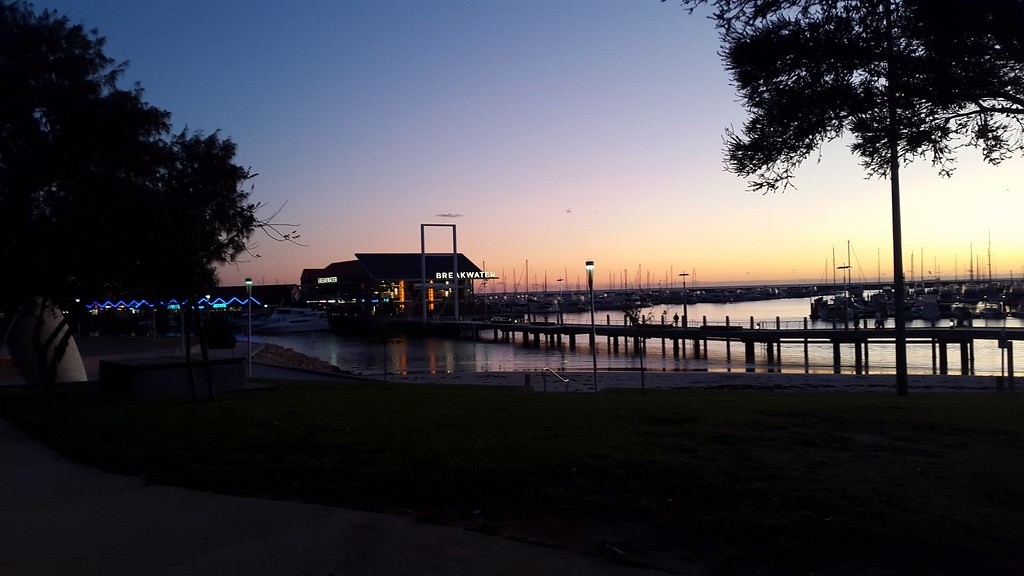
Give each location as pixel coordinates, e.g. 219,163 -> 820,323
557,279 -> 563,325
76,298 -> 81,344
679,273 -> 689,328
206,295 -> 211,320
245,279 -> 252,377
585,261 -> 598,391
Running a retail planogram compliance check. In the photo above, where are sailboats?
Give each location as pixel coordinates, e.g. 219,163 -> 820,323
473,260 -> 1024,322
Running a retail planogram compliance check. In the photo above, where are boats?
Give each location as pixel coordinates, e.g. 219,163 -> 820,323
238,307 -> 332,334
